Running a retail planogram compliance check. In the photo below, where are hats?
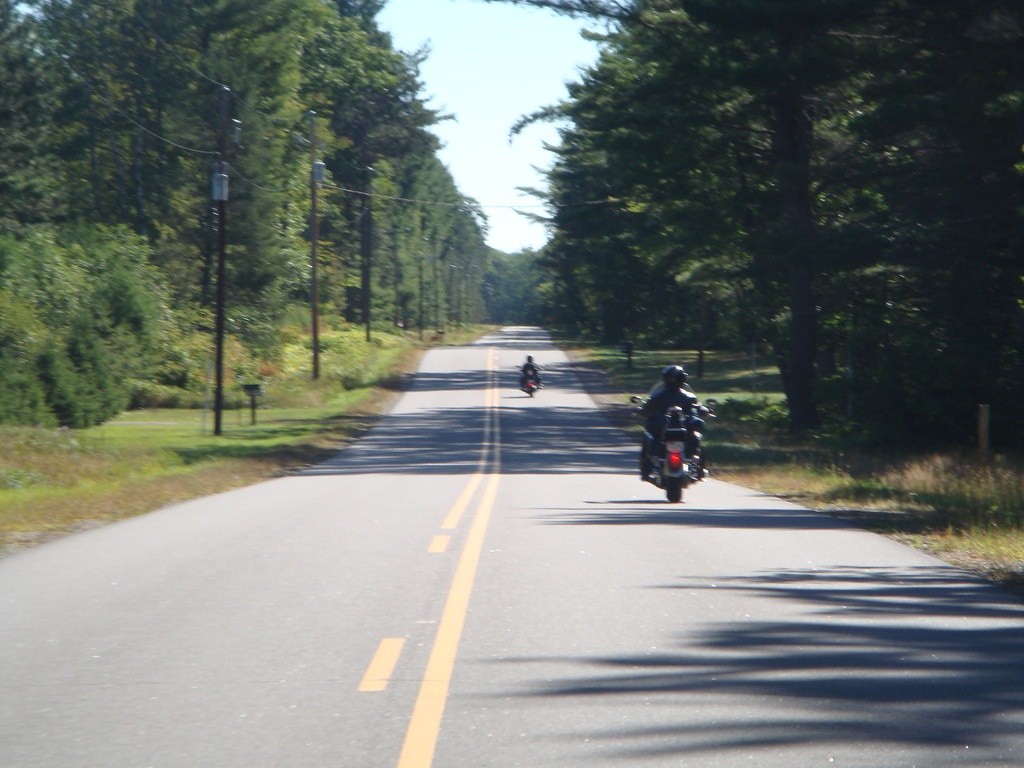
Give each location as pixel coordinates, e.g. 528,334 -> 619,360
662,366 -> 672,374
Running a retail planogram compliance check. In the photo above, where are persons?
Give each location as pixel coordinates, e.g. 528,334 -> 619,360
632,365 -> 714,483
519,355 -> 543,388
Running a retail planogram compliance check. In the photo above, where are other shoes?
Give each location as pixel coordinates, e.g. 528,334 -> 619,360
691,469 -> 709,477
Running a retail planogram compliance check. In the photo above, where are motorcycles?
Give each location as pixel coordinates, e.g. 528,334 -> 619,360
629,394 -> 718,506
516,362 -> 544,396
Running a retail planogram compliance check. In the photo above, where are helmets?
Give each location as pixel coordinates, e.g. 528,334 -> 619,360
527,356 -> 533,361
664,365 -> 686,386
